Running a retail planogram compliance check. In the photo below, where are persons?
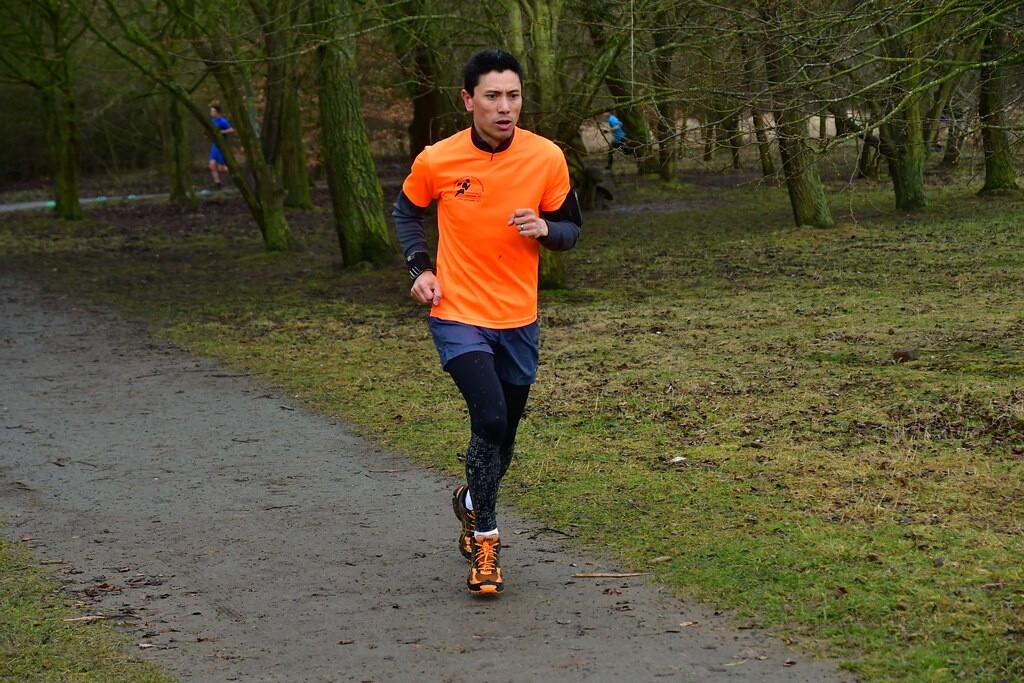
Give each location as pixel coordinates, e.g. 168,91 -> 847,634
392,50 -> 583,596
206,100 -> 237,192
601,109 -> 636,170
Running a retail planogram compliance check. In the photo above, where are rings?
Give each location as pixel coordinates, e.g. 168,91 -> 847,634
520,224 -> 523,229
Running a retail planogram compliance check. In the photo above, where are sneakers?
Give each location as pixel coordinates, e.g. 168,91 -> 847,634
451,484 -> 477,560
466,534 -> 505,595
